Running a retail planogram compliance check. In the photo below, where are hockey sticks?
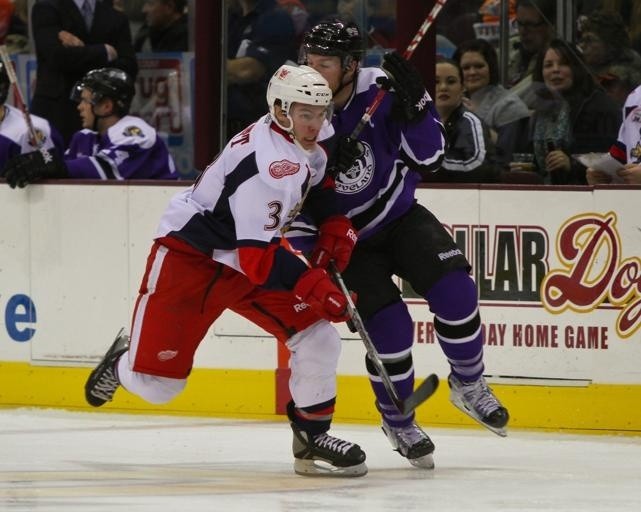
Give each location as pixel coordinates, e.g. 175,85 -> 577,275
331,257 -> 440,416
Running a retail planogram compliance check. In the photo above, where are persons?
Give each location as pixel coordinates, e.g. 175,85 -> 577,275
84,62 -> 366,468
1,0 -> 640,187
296,12 -> 510,460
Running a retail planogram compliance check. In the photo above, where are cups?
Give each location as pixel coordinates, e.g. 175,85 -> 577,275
513,153 -> 535,164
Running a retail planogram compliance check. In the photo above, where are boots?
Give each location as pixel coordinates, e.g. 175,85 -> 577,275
449,360 -> 509,428
86,334 -> 130,407
286,398 -> 367,467
375,399 -> 434,458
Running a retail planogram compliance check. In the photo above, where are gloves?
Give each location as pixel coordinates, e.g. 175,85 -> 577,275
2,146 -> 69,188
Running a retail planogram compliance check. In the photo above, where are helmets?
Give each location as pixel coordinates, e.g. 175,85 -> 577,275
71,68 -> 135,114
266,62 -> 335,133
297,20 -> 363,76
